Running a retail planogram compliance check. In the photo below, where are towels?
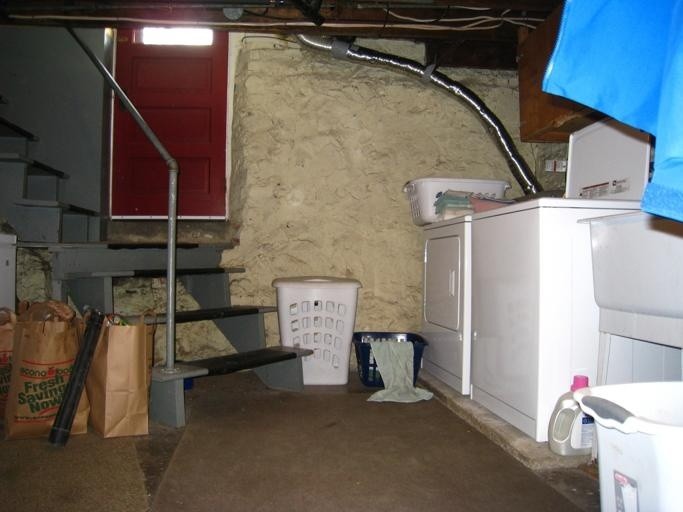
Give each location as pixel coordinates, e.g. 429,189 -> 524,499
367,341 -> 434,403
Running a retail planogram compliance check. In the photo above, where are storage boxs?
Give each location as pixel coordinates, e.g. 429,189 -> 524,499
573,382 -> 683,512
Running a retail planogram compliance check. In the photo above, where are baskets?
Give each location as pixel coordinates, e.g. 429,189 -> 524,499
352,330 -> 428,388
270,275 -> 361,386
402,178 -> 512,228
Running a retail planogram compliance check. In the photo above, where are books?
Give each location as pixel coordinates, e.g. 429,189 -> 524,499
433,189 -> 474,222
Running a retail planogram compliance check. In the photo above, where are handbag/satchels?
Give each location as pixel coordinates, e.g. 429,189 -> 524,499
0,303 -> 156,440
